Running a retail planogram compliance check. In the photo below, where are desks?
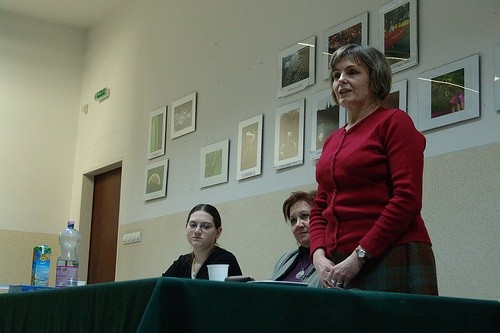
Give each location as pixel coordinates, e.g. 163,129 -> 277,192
0,277 -> 500,333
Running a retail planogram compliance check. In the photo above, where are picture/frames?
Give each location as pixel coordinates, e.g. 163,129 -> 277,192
378,0 -> 419,75
310,88 -> 345,161
144,158 -> 169,201
276,35 -> 316,99
494,44 -> 500,111
147,106 -> 167,160
273,97 -> 305,170
322,11 -> 369,81
383,79 -> 408,114
416,53 -> 480,131
236,113 -> 263,181
199,138 -> 229,188
170,91 -> 197,140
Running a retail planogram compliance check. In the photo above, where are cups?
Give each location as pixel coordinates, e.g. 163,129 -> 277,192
207,264 -> 230,281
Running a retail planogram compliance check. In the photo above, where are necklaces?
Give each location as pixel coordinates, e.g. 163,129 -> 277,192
296,257 -> 312,279
193,258 -> 201,276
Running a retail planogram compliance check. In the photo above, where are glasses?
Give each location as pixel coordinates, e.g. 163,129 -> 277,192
188,222 -> 216,231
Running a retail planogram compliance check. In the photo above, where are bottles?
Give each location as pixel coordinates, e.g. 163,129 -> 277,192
55,221 -> 81,289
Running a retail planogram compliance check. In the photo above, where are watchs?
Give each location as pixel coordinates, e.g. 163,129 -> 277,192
354,248 -> 369,262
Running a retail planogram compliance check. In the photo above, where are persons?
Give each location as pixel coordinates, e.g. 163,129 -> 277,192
271,191 -> 325,288
308,43 -> 439,295
161,204 -> 242,281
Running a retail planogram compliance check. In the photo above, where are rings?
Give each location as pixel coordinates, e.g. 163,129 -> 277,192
337,281 -> 343,285
331,280 -> 336,284
